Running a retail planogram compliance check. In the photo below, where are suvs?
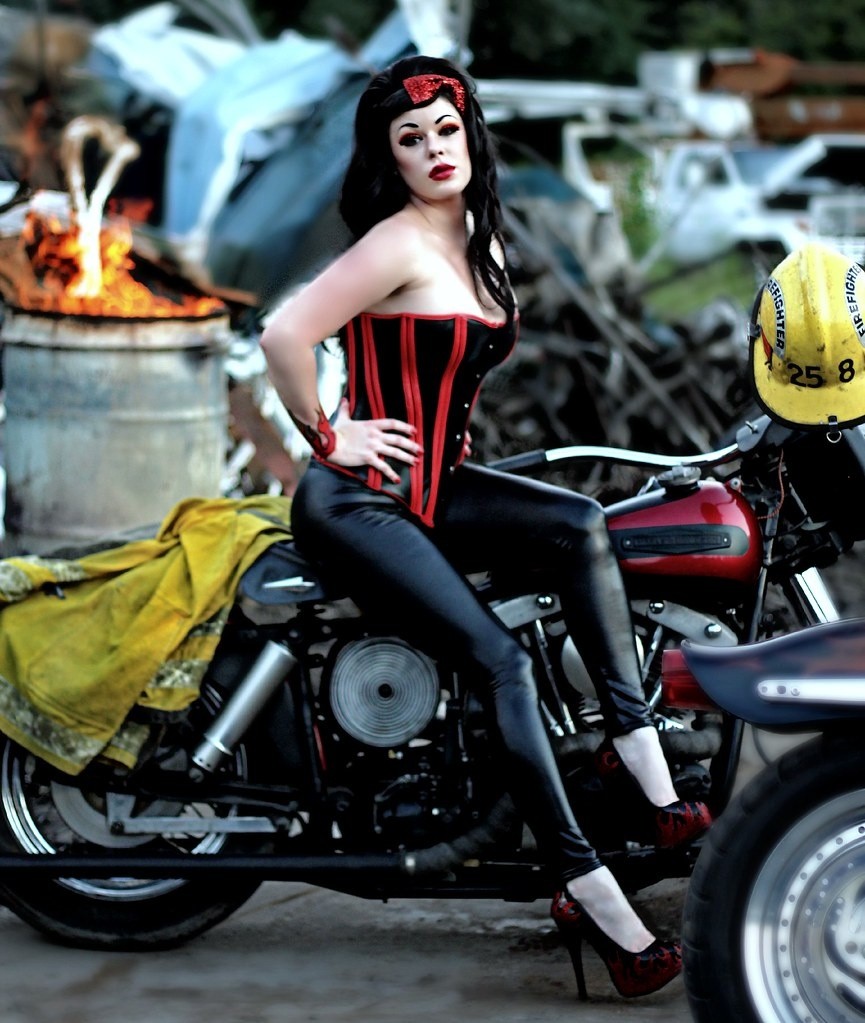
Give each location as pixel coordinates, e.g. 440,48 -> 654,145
564,125 -> 865,270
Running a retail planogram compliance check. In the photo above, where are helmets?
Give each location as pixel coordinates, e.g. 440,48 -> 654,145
748,241 -> 865,431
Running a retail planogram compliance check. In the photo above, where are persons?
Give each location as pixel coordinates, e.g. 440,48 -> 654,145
260,54 -> 712,1001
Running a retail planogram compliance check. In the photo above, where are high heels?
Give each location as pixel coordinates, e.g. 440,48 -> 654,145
592,737 -> 711,852
549,885 -> 684,999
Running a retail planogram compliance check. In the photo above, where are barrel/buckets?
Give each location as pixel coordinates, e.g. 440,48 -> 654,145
0,310 -> 230,550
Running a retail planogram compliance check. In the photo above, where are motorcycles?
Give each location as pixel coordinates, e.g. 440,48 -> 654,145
0,365 -> 865,1001
659,619 -> 865,1023
